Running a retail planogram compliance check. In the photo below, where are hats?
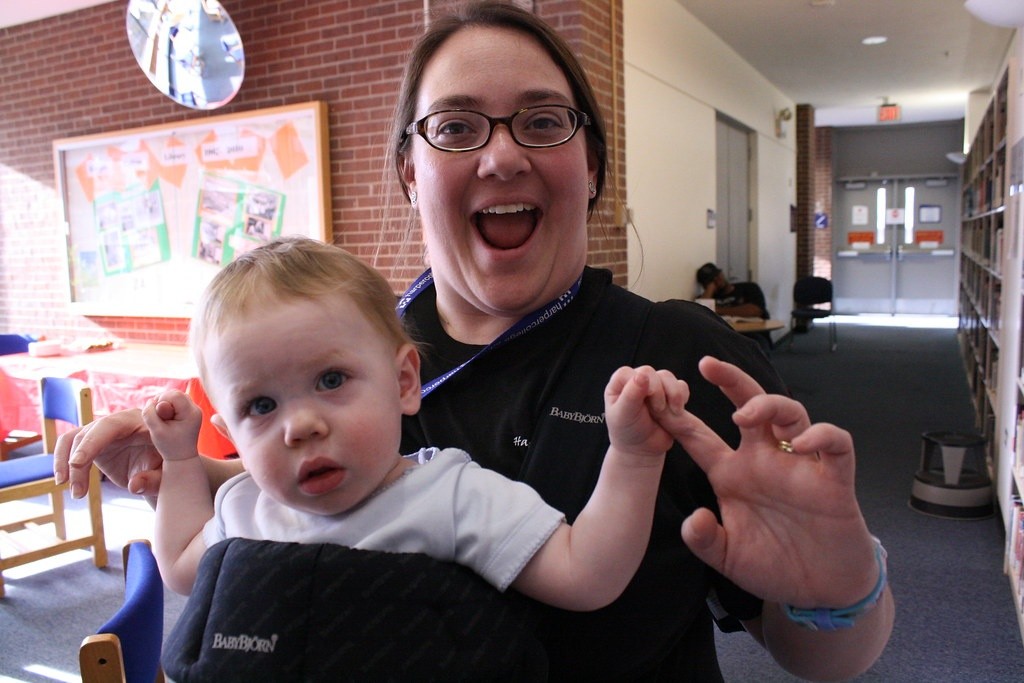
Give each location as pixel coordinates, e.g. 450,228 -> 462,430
697,264 -> 722,286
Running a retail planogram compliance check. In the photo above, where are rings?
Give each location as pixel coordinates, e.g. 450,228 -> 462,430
777,439 -> 805,454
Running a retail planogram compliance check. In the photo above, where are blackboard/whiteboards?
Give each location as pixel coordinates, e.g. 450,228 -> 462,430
53,102 -> 333,318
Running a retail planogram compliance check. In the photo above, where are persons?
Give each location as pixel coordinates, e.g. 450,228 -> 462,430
53,4 -> 895,679
694,263 -> 771,351
142,236 -> 691,680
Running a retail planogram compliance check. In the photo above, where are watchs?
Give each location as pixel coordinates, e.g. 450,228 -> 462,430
780,534 -> 891,632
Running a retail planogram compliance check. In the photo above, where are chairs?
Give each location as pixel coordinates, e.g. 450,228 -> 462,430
77,538 -> 169,682
0,375 -> 107,598
789,276 -> 838,351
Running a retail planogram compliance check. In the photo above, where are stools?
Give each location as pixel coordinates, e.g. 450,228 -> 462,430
908,426 -> 994,518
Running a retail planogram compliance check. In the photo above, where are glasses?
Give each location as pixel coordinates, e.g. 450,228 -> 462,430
400,104 -> 592,153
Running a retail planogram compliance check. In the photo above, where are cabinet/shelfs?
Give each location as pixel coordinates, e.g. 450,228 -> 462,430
954,55 -> 1024,650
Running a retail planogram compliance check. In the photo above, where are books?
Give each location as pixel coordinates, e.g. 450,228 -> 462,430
954,93 -> 1024,638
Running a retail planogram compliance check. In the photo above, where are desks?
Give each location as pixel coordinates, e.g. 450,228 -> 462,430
731,316 -> 787,333
